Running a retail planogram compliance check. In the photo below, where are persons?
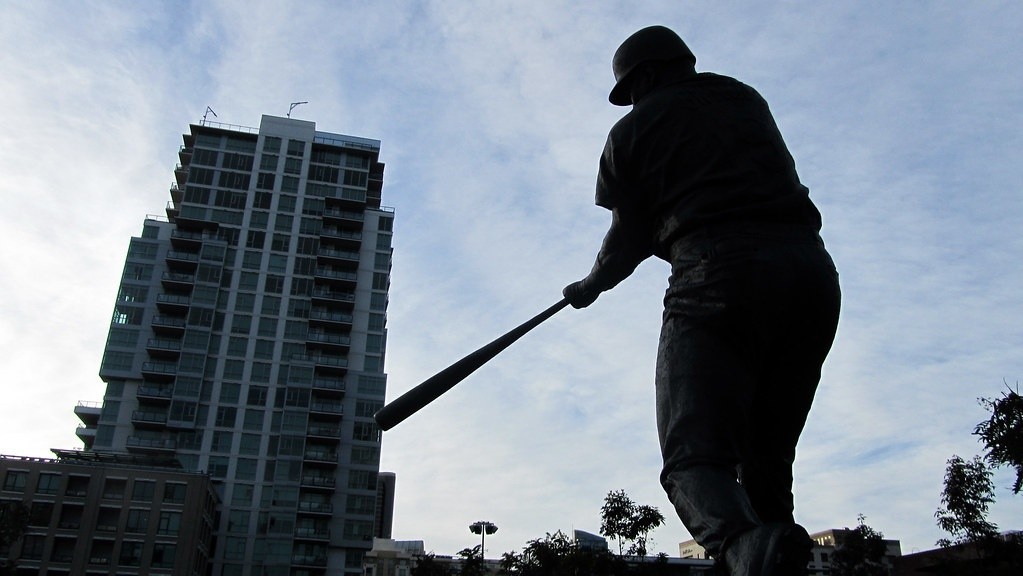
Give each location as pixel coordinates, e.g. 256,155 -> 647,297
564,26 -> 841,575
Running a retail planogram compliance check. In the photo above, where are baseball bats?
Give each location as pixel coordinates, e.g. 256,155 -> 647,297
371,296 -> 570,432
